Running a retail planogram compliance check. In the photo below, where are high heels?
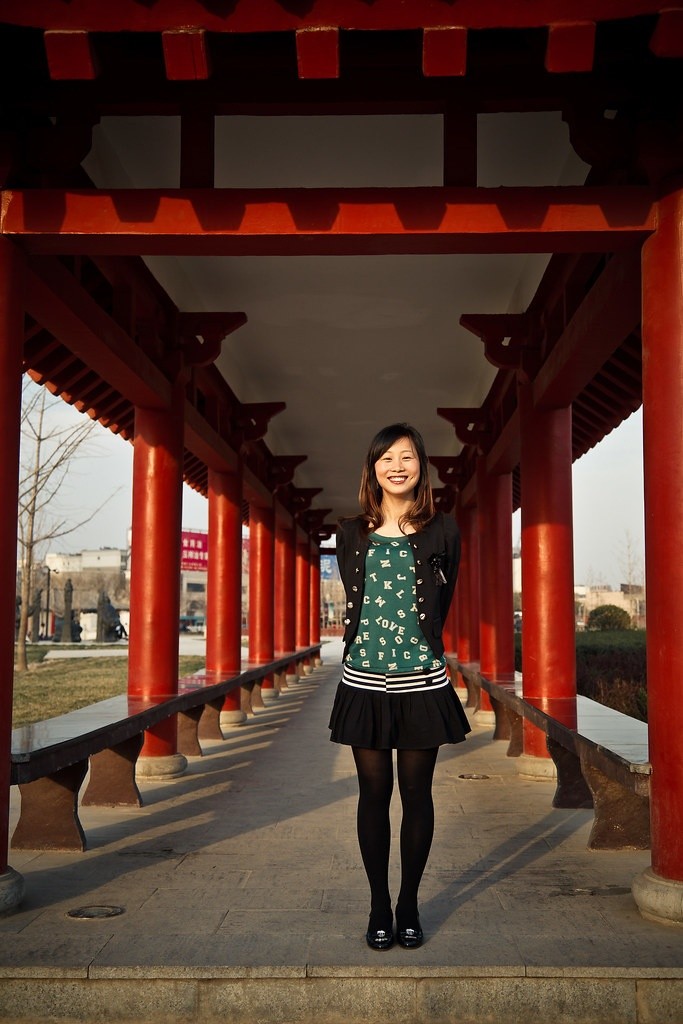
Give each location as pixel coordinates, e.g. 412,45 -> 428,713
366,908 -> 394,951
395,905 -> 423,949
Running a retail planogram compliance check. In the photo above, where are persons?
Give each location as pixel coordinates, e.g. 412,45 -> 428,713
326,421 -> 471,953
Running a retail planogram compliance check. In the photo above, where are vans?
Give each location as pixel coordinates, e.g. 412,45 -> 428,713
180,615 -> 204,634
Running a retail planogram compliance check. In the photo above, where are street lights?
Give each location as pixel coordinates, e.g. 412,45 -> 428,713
42,565 -> 55,642
